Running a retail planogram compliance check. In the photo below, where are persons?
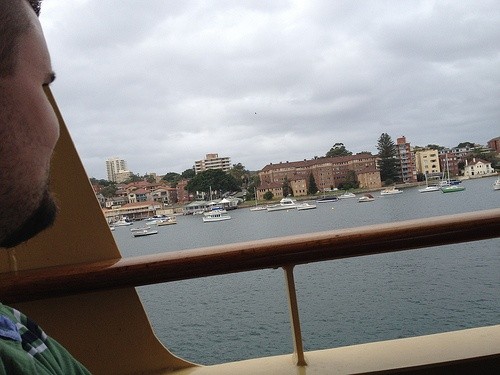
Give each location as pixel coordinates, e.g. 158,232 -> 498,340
0,0 -> 92,375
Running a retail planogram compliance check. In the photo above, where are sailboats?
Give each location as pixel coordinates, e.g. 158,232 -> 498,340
315,168 -> 337,203
437,152 -> 463,188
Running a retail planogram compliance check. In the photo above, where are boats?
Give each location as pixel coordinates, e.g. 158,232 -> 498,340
380,187 -> 403,196
358,196 -> 375,203
296,203 -> 317,211
337,191 -> 356,200
249,186 -> 297,212
418,186 -> 440,193
130,226 -> 158,237
182,204 -> 228,216
113,215 -> 133,226
491,176 -> 500,191
202,212 -> 231,222
440,185 -> 465,193
109,225 -> 115,231
144,214 -> 177,226
